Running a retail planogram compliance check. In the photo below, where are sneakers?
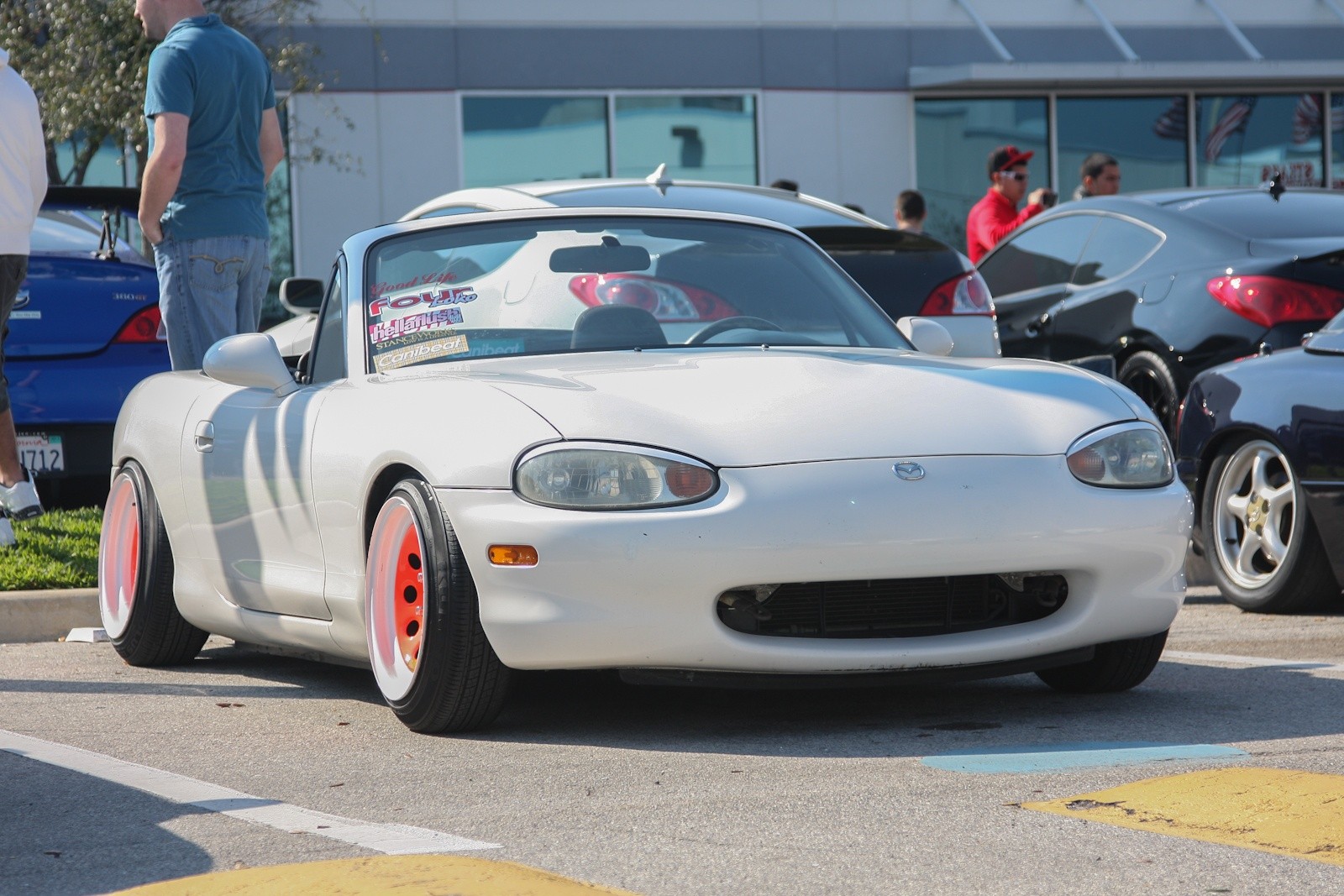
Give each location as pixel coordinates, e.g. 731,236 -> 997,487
0,463 -> 45,519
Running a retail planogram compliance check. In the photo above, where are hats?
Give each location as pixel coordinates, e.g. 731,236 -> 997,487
985,144 -> 1033,181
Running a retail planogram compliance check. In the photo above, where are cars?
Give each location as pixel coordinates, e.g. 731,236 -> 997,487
969,169 -> 1344,616
260,163 -> 1000,386
0,206 -> 176,518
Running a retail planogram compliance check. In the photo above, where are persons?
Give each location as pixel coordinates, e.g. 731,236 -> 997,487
893,189 -> 927,234
1,49 -> 45,554
771,178 -> 800,192
130,0 -> 285,366
1071,150 -> 1121,200
964,146 -> 1054,263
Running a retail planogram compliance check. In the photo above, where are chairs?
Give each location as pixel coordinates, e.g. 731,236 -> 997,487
567,305 -> 669,352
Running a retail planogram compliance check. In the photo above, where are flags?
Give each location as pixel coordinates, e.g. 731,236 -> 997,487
1151,91 -> 1342,160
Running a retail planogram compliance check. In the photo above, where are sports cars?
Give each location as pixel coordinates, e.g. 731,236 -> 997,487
100,203 -> 1197,734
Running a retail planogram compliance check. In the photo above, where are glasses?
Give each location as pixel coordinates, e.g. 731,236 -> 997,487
998,170 -> 1029,181
